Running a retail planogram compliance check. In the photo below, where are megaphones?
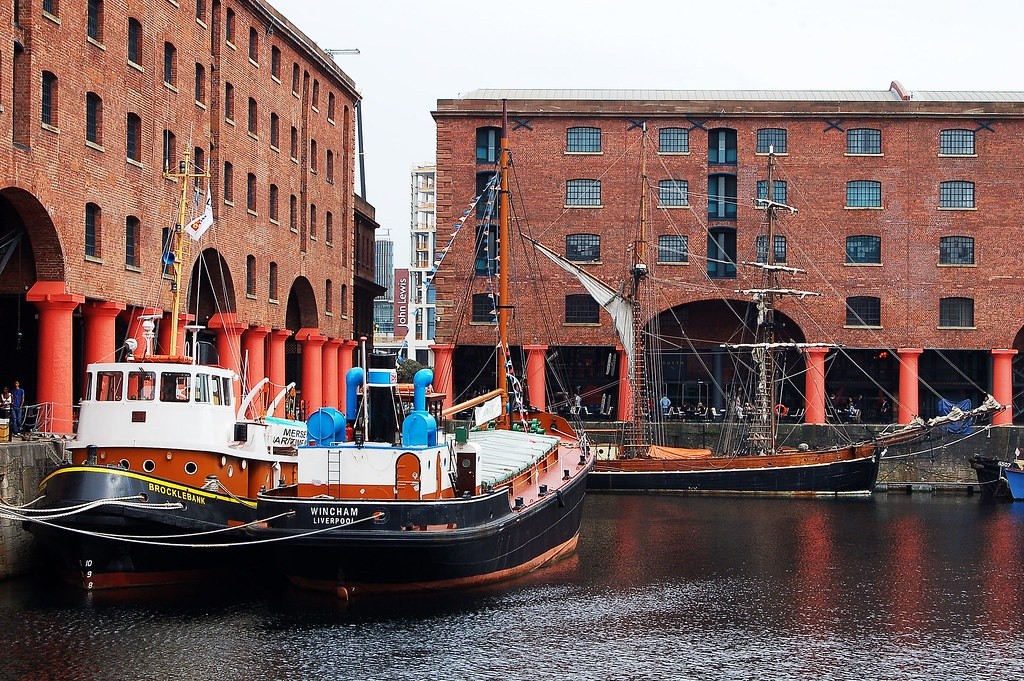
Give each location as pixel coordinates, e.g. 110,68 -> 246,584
164,252 -> 176,264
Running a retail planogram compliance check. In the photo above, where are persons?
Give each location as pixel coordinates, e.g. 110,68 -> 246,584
0,379 -> 24,436
660,394 -> 671,422
563,392 -> 582,413
879,397 -> 890,424
827,394 -> 862,424
680,402 -> 705,422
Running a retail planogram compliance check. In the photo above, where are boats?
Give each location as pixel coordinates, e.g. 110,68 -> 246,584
968,450 -> 1024,501
19,119 -> 308,606
241,98 -> 598,605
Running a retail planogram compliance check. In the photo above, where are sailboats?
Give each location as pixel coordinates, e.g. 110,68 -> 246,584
518,121 -> 1012,497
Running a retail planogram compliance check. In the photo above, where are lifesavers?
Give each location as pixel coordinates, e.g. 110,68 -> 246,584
774,402 -> 787,417
557,490 -> 567,507
597,448 -> 604,454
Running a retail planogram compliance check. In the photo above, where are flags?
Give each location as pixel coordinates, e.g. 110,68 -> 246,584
184,189 -> 213,242
475,395 -> 502,427
